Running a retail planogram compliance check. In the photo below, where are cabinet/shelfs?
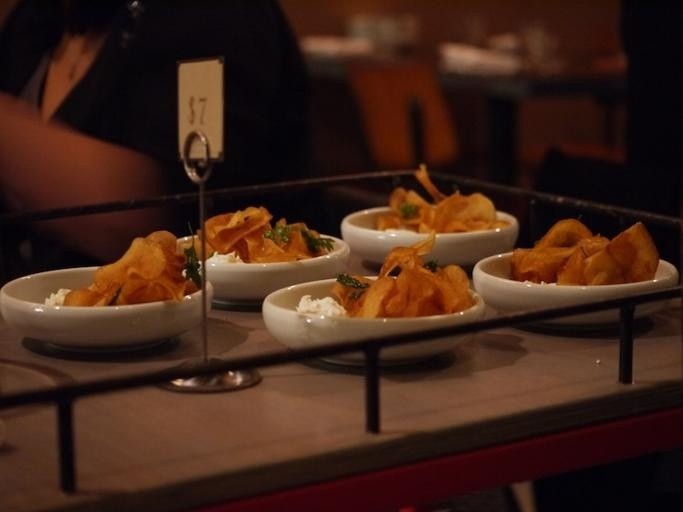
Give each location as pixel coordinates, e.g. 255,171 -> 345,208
0,214 -> 682,509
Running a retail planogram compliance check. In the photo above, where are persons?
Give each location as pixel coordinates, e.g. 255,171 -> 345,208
0,0 -> 314,272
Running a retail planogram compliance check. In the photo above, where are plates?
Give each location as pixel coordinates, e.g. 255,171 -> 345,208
261,275 -> 484,362
205,235 -> 350,300
0,267 -> 214,348
472,251 -> 636,324
341,205 -> 520,267
